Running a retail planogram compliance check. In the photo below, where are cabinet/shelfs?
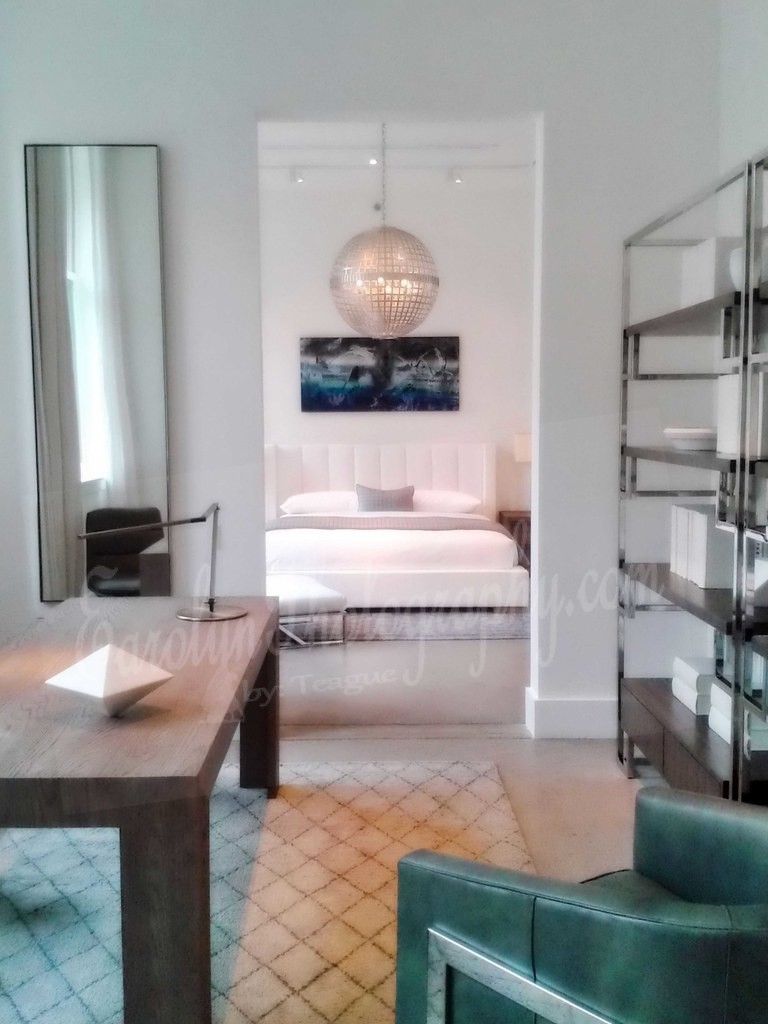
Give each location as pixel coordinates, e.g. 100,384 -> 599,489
614,147 -> 768,808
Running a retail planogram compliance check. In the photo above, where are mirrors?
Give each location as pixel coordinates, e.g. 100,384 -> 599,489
23,143 -> 172,602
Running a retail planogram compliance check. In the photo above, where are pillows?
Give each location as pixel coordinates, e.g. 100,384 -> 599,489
279,491 -> 358,514
355,484 -> 414,511
412,490 -> 481,513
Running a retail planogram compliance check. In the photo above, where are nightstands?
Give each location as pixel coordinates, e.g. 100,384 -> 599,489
499,511 -> 531,562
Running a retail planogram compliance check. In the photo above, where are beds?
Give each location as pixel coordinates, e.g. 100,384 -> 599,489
266,443 -> 531,641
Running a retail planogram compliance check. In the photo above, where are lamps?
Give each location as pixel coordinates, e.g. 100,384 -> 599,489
329,124 -> 439,340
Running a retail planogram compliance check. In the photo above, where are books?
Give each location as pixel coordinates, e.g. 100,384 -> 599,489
671,657 -> 768,751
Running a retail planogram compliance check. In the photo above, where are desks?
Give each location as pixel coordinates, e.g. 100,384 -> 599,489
0,597 -> 280,1024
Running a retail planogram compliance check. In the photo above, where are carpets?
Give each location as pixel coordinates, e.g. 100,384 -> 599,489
0,761 -> 539,1024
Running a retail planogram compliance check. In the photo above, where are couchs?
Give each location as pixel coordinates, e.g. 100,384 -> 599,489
395,786 -> 768,1024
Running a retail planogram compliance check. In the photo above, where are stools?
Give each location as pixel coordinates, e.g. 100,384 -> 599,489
266,574 -> 349,650
